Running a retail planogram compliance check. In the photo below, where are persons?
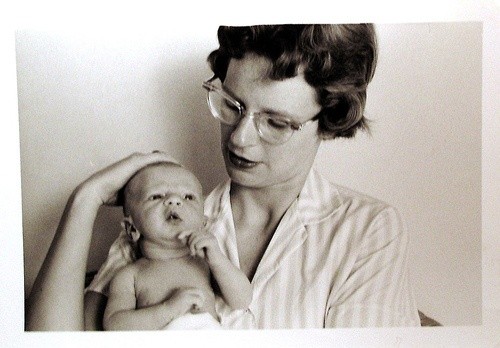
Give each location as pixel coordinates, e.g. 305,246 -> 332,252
101,160 -> 253,330
25,24 -> 422,332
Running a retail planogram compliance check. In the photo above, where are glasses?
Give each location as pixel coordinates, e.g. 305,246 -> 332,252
201,74 -> 322,145
